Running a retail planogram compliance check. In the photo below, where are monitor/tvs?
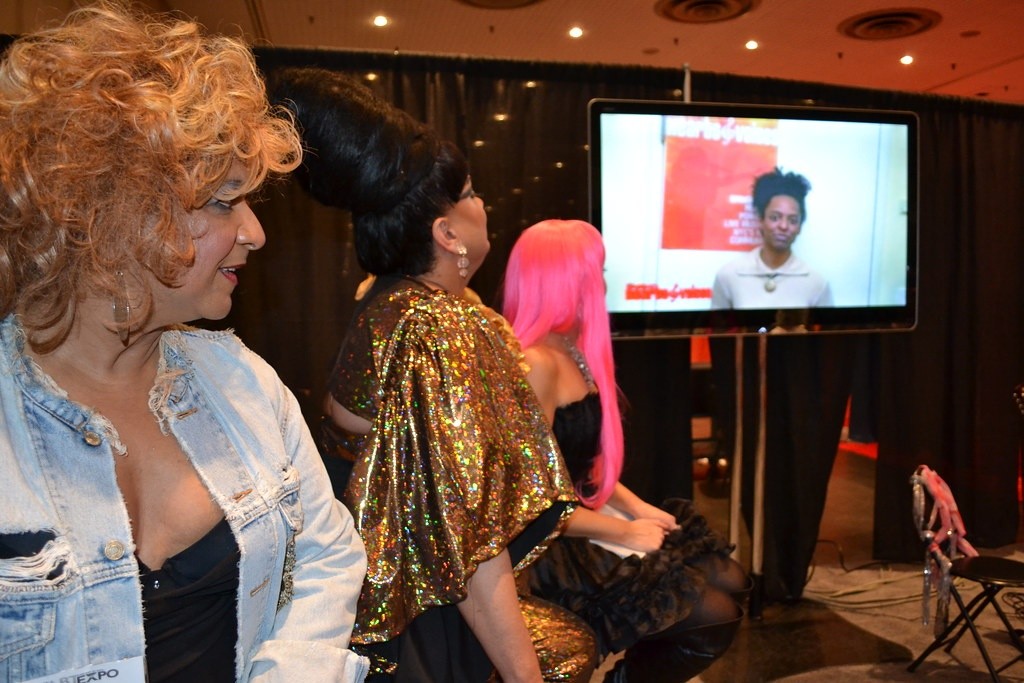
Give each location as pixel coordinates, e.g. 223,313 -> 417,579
586,96 -> 921,341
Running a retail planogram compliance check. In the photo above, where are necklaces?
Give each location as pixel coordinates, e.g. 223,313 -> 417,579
560,334 -> 594,395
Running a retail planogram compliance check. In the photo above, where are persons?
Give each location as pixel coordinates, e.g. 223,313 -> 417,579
505,218 -> 749,683
0,2 -> 369,683
710,165 -> 831,310
272,68 -> 597,683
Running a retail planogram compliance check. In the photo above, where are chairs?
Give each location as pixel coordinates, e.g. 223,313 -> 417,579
906,463 -> 1024,683
